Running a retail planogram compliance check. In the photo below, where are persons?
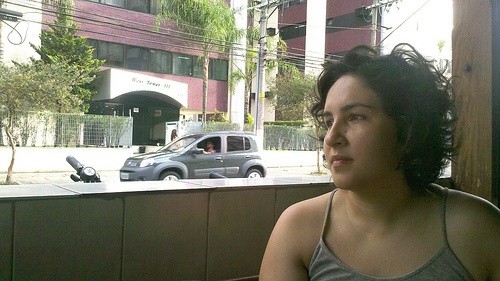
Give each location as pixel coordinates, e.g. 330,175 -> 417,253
171,129 -> 178,141
259,43 -> 500,281
203,140 -> 216,155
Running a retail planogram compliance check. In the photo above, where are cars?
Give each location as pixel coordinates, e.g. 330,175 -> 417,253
119,130 -> 268,182
